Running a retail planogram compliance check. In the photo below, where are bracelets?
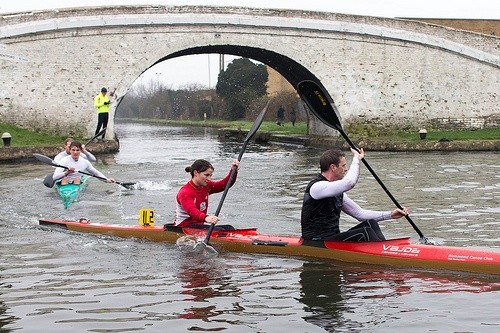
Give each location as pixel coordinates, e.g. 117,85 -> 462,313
204,215 -> 209,223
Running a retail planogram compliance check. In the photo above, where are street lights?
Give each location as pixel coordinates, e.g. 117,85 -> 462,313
156,72 -> 162,88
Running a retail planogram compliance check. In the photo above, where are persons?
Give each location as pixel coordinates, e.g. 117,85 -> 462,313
290,108 -> 296,126
301,147 -> 409,249
52,138 -> 96,170
95,87 -> 111,141
175,158 -> 240,231
52,141 -> 114,188
277,105 -> 286,126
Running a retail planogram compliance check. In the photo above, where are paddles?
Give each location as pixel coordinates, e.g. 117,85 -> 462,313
81,128 -> 106,149
33,152 -> 136,188
198,99 -> 273,249
296,80 -> 423,238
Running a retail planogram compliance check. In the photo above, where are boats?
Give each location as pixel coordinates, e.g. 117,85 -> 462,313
52,173 -> 92,209
38,209 -> 500,276
